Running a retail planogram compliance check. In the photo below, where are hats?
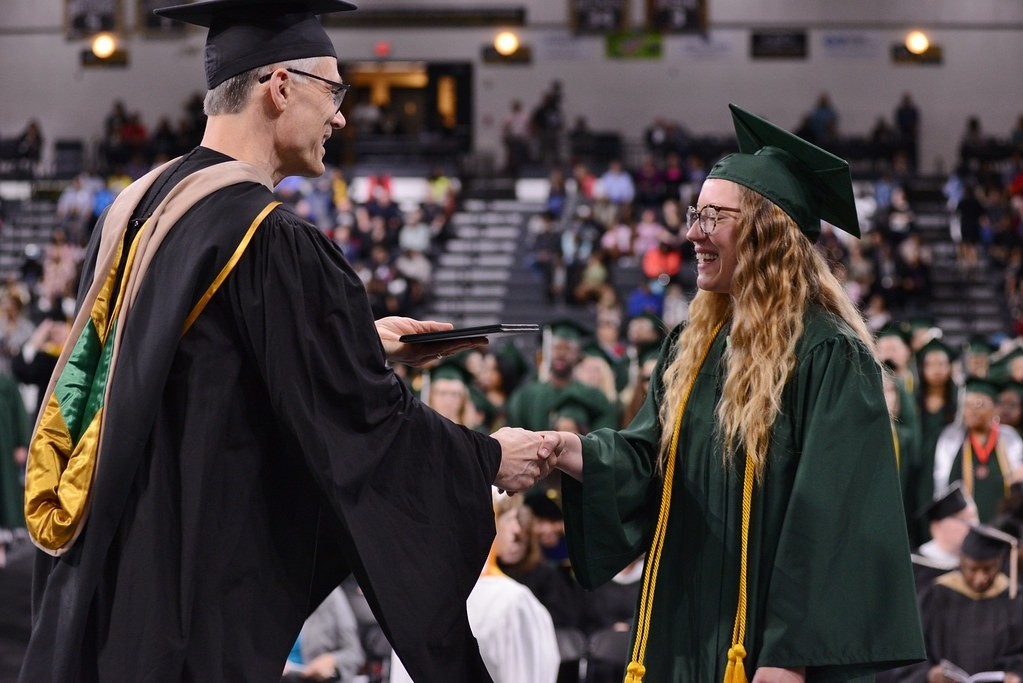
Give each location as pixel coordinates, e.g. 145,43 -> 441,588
961,523 -> 1019,600
154,0 -> 359,90
880,318 -> 1021,526
522,487 -> 565,523
706,103 -> 861,245
413,314 -> 663,426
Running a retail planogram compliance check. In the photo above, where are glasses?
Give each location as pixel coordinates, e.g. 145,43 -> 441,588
259,68 -> 350,114
686,204 -> 741,234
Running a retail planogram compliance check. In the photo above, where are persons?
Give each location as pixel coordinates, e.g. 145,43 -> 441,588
500,102 -> 934,683
16,1 -> 565,683
0,83 -> 1023,683
